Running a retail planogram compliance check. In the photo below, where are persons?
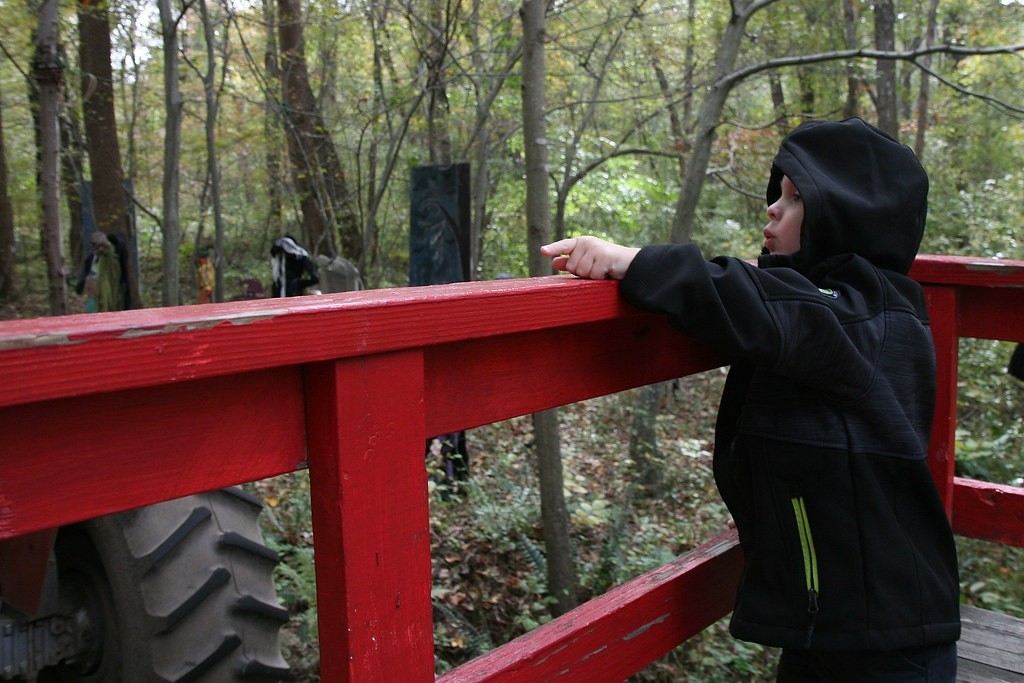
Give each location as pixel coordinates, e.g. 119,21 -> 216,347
541,116 -> 961,683
